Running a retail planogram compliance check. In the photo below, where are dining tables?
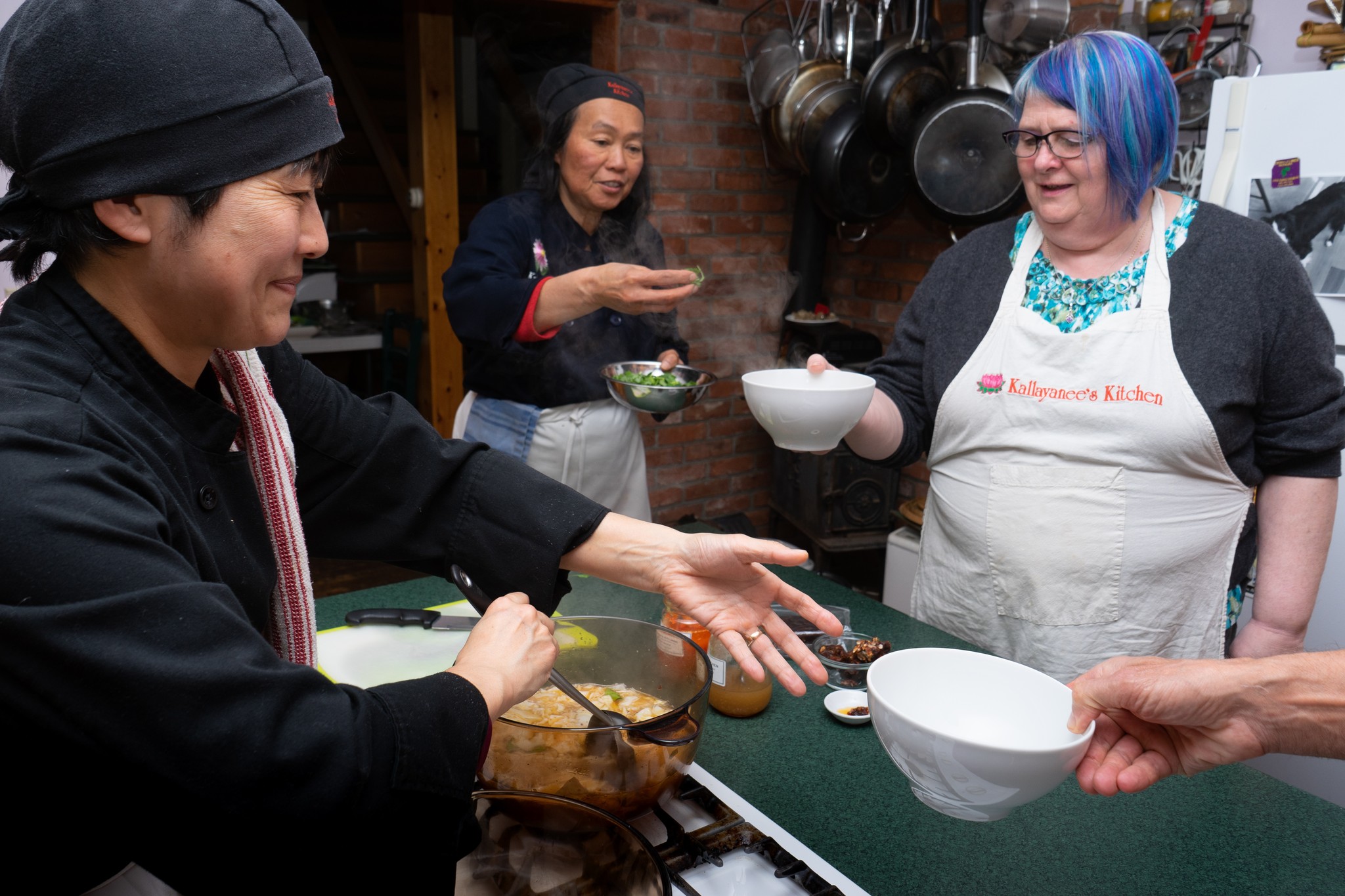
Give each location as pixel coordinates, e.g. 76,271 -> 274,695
311,522 -> 1345,896
284,313 -> 384,357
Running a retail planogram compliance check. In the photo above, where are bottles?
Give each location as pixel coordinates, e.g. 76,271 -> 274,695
1186,34 -> 1199,66
706,633 -> 773,718
656,597 -> 713,683
1171,0 -> 1200,19
1201,37 -> 1230,66
1151,43 -> 1167,62
1167,43 -> 1186,73
1147,0 -> 1172,22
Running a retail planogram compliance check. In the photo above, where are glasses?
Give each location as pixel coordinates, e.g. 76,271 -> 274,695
1002,129 -> 1102,159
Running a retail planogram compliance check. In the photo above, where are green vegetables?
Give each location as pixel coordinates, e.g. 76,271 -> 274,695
606,368 -> 701,387
677,264 -> 705,289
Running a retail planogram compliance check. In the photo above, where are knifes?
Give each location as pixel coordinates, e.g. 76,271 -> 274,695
344,608 -> 482,631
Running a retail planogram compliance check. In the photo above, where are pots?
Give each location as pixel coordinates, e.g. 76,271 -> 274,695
731,0 -> 1070,246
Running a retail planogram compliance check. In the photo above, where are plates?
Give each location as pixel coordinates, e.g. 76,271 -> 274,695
785,314 -> 839,324
285,325 -> 323,338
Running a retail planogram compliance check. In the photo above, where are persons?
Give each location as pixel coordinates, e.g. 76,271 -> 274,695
790,29 -> 1343,684
0,2 -> 846,896
1066,648 -> 1345,800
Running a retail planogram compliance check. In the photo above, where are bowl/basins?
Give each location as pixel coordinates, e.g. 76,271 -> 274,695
450,792 -> 672,896
865,647 -> 1097,822
823,689 -> 871,724
811,631 -> 886,691
451,616 -> 714,834
600,361 -> 717,414
764,604 -> 855,658
741,368 -> 876,451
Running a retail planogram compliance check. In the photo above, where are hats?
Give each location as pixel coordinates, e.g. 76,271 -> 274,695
0,0 -> 346,242
536,63 -> 645,129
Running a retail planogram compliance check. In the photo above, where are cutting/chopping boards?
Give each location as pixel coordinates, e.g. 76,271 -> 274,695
315,599 -> 599,689
